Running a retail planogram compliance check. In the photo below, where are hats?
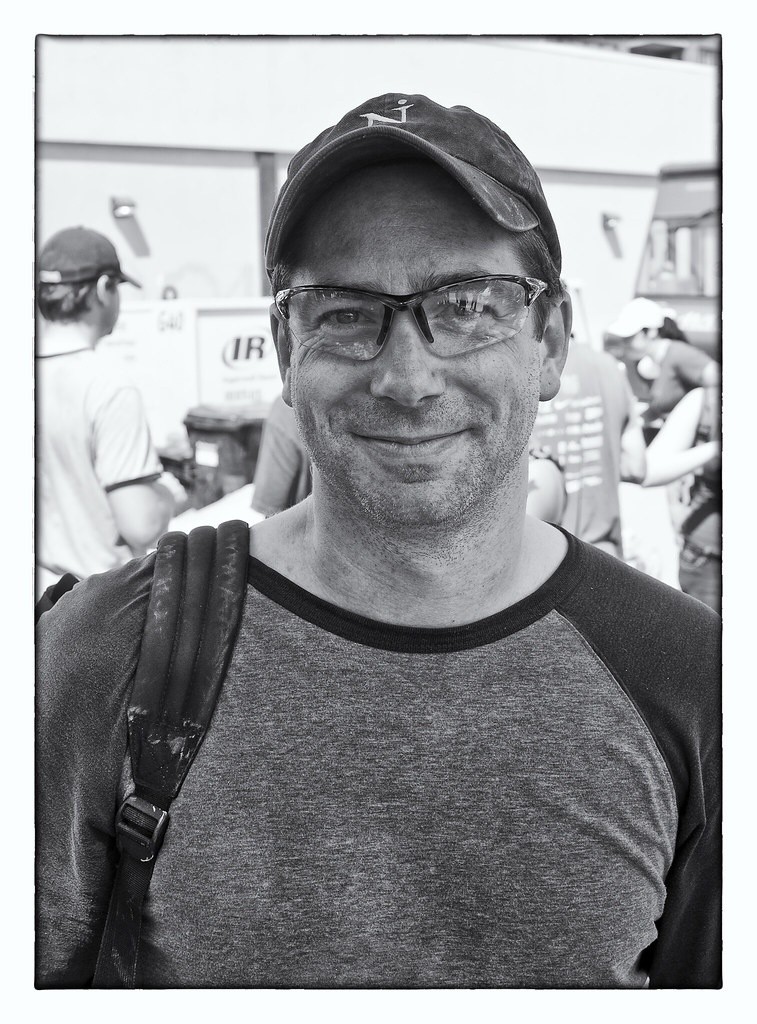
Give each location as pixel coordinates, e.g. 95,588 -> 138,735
604,297 -> 666,339
36,227 -> 142,289
266,93 -> 561,287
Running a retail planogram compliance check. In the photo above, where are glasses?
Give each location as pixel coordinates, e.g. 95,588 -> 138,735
274,273 -> 553,363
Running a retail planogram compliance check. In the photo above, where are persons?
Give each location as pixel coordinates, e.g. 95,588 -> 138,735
34,93 -> 722,990
250,391 -> 314,519
605,296 -> 723,592
527,309 -> 648,562
35,225 -> 189,603
